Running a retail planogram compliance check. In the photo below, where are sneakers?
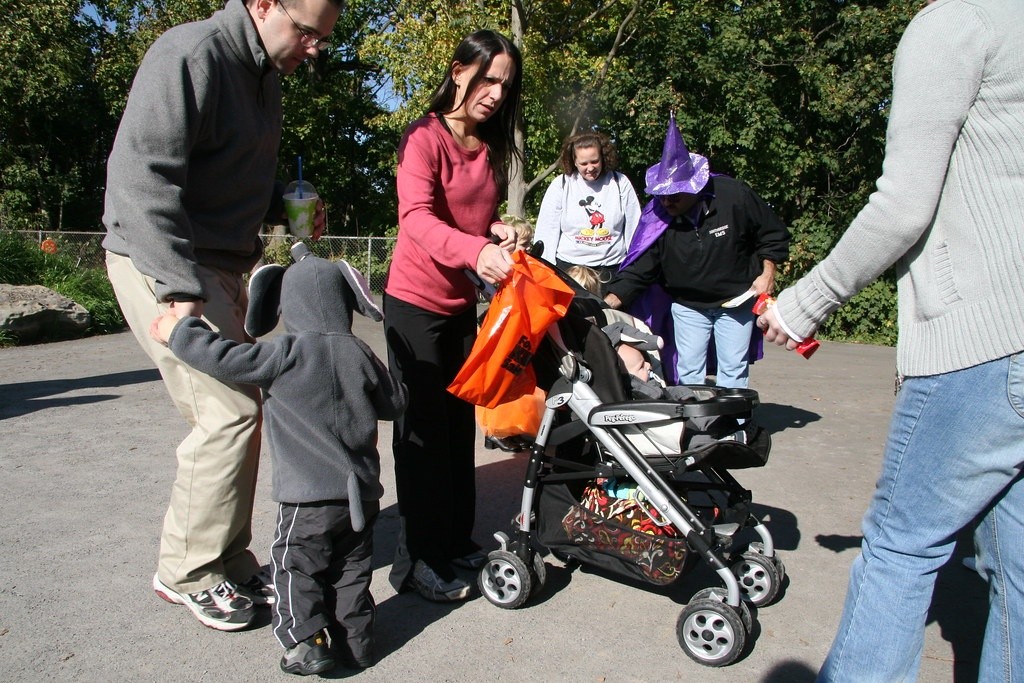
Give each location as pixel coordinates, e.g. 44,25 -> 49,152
151,567 -> 258,632
455,548 -> 495,580
344,647 -> 373,669
281,632 -> 338,674
407,561 -> 472,603
241,567 -> 279,609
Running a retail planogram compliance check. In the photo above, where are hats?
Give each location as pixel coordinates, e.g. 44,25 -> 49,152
639,110 -> 711,197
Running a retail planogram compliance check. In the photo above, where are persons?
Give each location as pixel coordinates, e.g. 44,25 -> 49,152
500,110 -> 791,454
158,254 -> 410,676
760,0 -> 1024,683
382,30 -> 528,605
101,0 -> 346,631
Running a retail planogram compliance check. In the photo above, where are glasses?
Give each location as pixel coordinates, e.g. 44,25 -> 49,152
278,1 -> 333,51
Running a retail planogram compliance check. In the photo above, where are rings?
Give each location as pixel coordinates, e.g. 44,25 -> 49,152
758,314 -> 768,326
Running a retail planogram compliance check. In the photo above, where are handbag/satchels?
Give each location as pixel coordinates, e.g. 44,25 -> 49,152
445,250 -> 575,408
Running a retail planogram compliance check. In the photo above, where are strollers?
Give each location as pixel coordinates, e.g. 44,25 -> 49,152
463,240 -> 785,667
